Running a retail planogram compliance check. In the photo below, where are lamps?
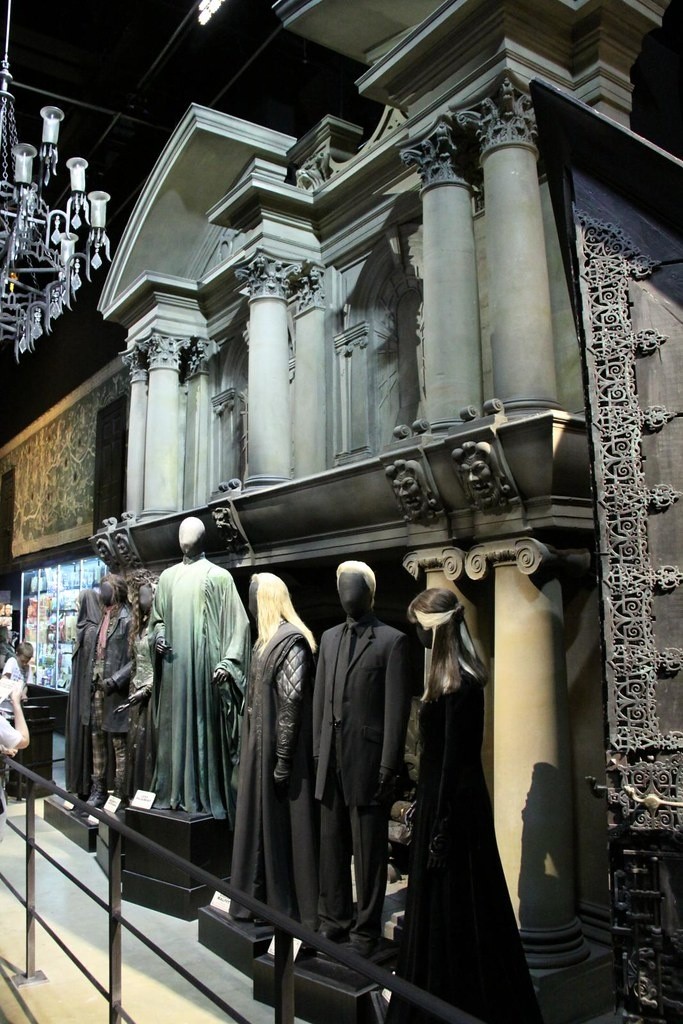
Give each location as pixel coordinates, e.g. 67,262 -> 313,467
0,0 -> 114,364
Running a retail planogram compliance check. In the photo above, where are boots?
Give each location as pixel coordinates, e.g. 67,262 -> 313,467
112,777 -> 130,810
86,775 -> 109,807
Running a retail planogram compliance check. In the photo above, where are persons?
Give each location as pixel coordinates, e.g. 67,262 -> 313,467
59,513 -> 550,1024
0,602 -> 34,814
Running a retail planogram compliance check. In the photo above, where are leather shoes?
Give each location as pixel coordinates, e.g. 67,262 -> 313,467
344,938 -> 383,958
302,932 -> 349,950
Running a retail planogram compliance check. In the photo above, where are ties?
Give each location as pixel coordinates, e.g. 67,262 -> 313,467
333,623 -> 354,722
97,610 -> 110,660
